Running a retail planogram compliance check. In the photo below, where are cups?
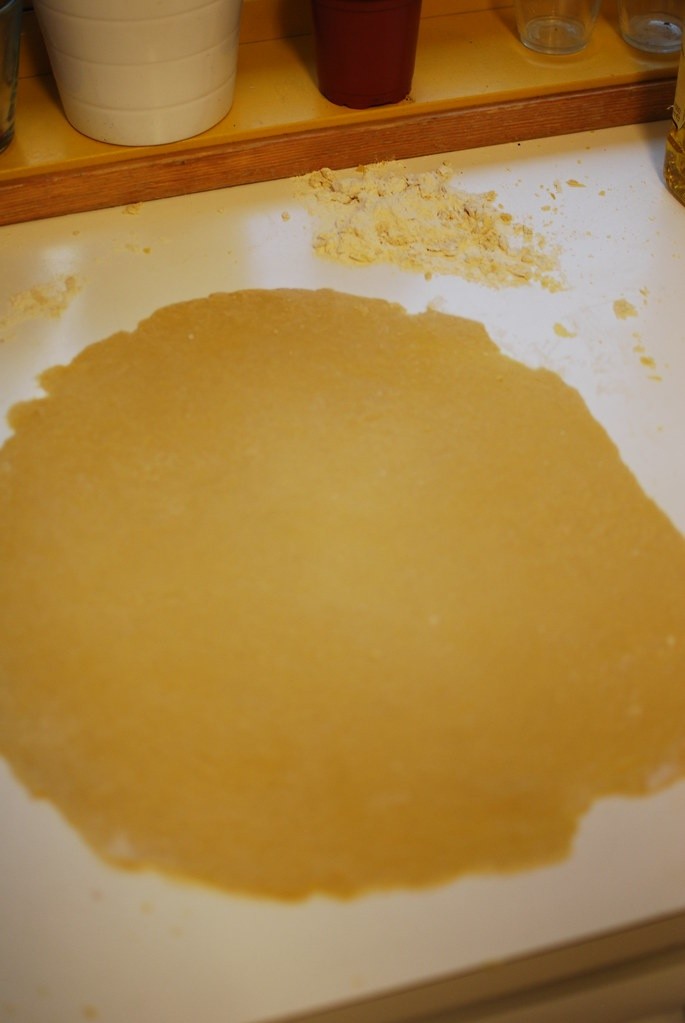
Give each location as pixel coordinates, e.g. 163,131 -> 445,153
512,0 -> 602,56
32,1 -> 243,146
311,0 -> 421,108
617,0 -> 685,53
0,1 -> 21,154
